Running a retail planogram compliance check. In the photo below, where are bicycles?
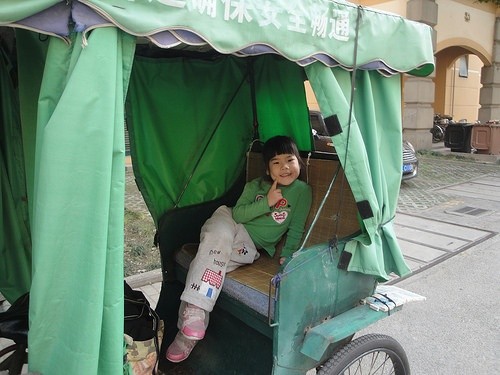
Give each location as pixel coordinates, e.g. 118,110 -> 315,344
432,113 -> 469,143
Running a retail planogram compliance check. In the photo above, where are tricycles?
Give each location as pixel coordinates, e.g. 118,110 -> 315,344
0,0 -> 435,375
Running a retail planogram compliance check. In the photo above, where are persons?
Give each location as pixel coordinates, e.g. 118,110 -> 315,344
166,135 -> 313,361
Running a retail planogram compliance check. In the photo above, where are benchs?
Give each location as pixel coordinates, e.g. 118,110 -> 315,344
176,139 -> 360,339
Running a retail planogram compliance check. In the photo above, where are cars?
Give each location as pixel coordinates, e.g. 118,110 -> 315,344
309,109 -> 418,180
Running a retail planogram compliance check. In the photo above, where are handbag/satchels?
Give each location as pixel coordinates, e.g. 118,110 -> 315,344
124,280 -> 159,341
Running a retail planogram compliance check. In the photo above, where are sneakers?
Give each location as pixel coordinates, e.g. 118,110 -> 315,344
179,303 -> 205,340
166,331 -> 198,362
